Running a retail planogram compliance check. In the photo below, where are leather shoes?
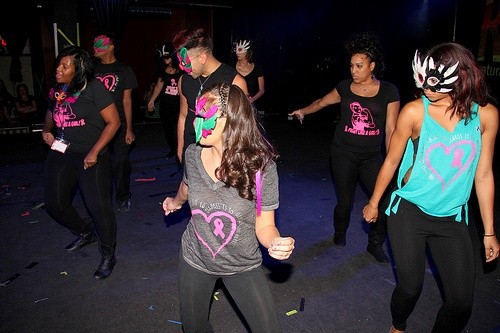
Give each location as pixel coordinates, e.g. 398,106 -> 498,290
94,241 -> 117,280
64,218 -> 98,251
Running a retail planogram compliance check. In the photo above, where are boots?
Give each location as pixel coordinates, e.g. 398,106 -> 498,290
367,211 -> 389,264
333,205 -> 351,249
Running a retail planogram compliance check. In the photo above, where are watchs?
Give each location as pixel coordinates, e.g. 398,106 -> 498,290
147,43 -> 188,170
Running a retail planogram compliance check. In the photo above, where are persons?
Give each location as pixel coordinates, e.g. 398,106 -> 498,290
173,27 -> 251,164
232,38 -> 265,120
0,80 -> 16,126
364,43 -> 500,333
14,83 -> 38,121
86,32 -> 137,213
41,45 -> 122,282
287,46 -> 400,266
163,83 -> 295,333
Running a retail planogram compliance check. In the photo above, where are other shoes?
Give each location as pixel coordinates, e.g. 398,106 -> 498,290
114,199 -> 131,215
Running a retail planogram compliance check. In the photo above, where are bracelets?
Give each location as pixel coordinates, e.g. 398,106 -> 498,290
482,233 -> 495,238
